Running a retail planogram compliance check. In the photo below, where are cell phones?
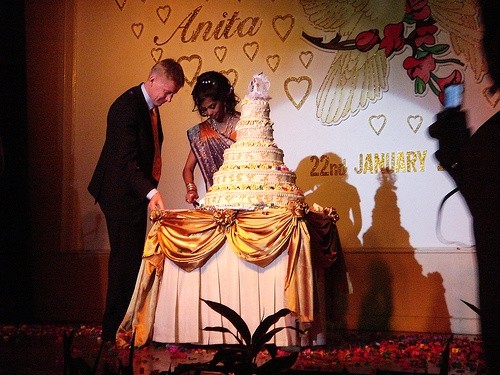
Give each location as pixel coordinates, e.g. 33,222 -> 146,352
444,82 -> 463,112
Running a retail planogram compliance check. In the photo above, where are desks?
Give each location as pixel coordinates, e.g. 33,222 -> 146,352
149,205 -> 340,352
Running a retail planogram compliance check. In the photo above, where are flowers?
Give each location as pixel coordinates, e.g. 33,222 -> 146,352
320,206 -> 340,223
213,209 -> 235,225
286,199 -> 310,219
299,0 -> 466,107
149,206 -> 164,223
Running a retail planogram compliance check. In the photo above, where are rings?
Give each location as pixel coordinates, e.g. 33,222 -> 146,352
189,194 -> 193,196
187,200 -> 189,202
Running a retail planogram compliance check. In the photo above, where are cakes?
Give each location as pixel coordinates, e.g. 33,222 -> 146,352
196,71 -> 305,210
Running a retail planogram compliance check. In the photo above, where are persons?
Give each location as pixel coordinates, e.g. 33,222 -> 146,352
182,71 -> 244,206
85,59 -> 185,338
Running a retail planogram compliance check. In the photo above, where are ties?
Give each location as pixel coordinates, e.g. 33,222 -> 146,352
151,107 -> 161,181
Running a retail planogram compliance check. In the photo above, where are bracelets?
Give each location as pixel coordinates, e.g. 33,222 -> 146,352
186,182 -> 197,193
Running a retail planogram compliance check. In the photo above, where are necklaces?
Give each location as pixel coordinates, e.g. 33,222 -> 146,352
210,111 -> 233,137
